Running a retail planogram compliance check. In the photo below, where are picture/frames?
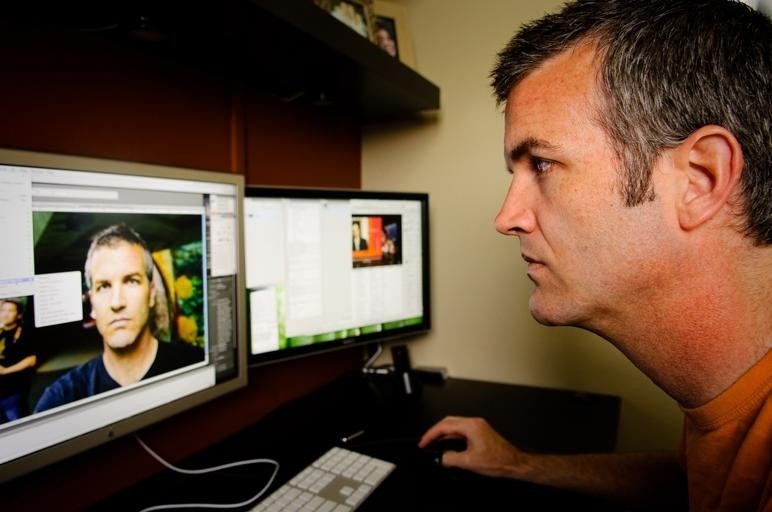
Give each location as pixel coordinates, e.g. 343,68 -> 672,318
369,15 -> 400,59
317,1 -> 378,42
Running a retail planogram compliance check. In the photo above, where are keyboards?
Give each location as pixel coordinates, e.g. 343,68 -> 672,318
246,443 -> 397,511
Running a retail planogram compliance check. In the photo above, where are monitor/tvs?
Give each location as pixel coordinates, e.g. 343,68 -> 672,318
0,147 -> 248,480
242,184 -> 432,364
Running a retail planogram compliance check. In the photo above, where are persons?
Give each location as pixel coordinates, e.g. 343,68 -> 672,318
0,299 -> 39,423
353,220 -> 369,250
33,220 -> 205,416
415,0 -> 772,512
379,227 -> 396,265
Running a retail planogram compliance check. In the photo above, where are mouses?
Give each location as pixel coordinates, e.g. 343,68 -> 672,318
410,437 -> 467,469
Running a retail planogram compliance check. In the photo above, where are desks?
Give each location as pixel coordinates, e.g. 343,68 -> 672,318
102,369 -> 621,512
